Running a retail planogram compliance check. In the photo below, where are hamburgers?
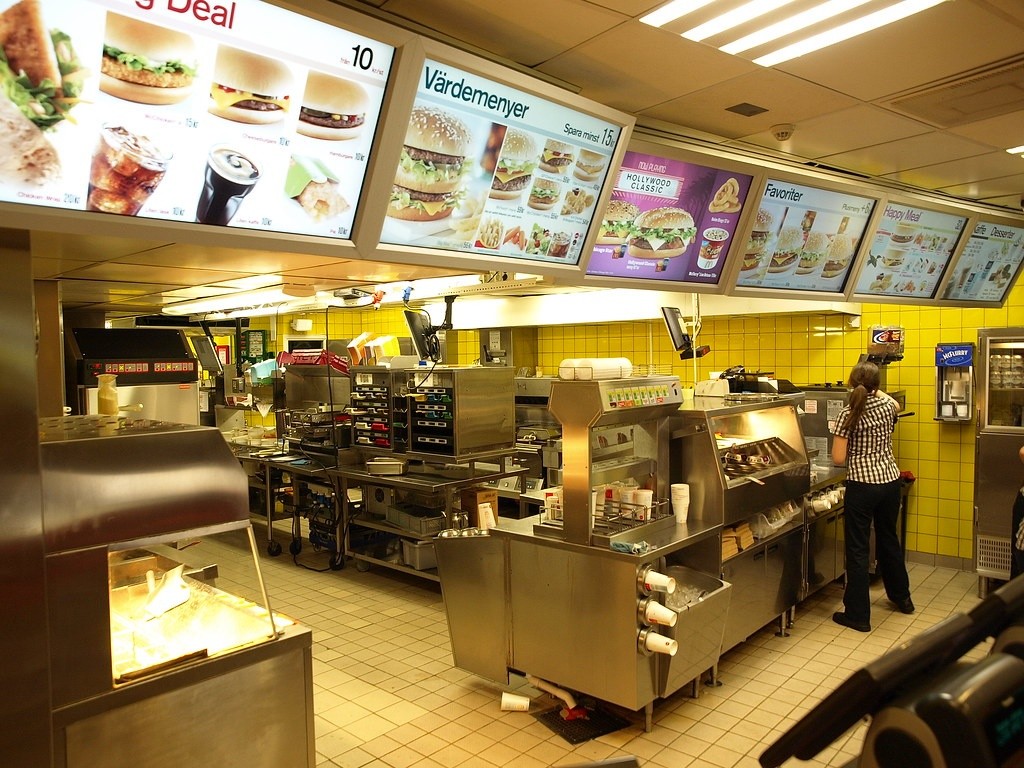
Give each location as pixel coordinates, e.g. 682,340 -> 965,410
735,208 -> 853,278
880,225 -> 918,272
206,44 -> 296,125
573,149 -> 606,182
489,129 -> 540,199
527,177 -> 562,210
294,70 -> 371,140
539,138 -> 574,173
386,105 -> 472,222
99,10 -> 198,105
594,200 -> 697,259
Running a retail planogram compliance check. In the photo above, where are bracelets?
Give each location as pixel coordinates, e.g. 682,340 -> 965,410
874,388 -> 879,397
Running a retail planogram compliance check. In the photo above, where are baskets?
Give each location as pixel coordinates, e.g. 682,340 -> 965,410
385,502 -> 469,539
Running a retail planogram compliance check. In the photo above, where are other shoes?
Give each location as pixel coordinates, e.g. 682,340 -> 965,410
832,611 -> 871,632
897,596 -> 915,614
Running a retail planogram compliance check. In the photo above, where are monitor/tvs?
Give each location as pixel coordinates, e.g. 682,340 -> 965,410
404,310 -> 430,361
661,307 -> 692,352
190,336 -> 223,371
759,573 -> 1024,768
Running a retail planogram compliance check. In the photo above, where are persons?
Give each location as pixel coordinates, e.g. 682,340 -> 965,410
1011,445 -> 1024,581
831,362 -> 915,633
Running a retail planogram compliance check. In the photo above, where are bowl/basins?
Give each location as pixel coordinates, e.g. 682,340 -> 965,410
220,432 -> 277,446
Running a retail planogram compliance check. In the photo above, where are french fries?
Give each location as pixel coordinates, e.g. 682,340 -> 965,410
480,217 -> 503,246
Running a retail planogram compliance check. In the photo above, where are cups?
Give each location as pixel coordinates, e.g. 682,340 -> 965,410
545,481 -> 653,529
810,486 -> 846,513
671,482 -> 691,524
500,691 -> 530,711
645,631 -> 678,657
696,227 -> 729,270
644,570 -> 677,595
643,601 -> 678,628
85,120 -> 174,216
477,502 -> 496,531
195,144 -> 261,227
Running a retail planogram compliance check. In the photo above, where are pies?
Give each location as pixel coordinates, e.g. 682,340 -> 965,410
0,0 -> 86,189
